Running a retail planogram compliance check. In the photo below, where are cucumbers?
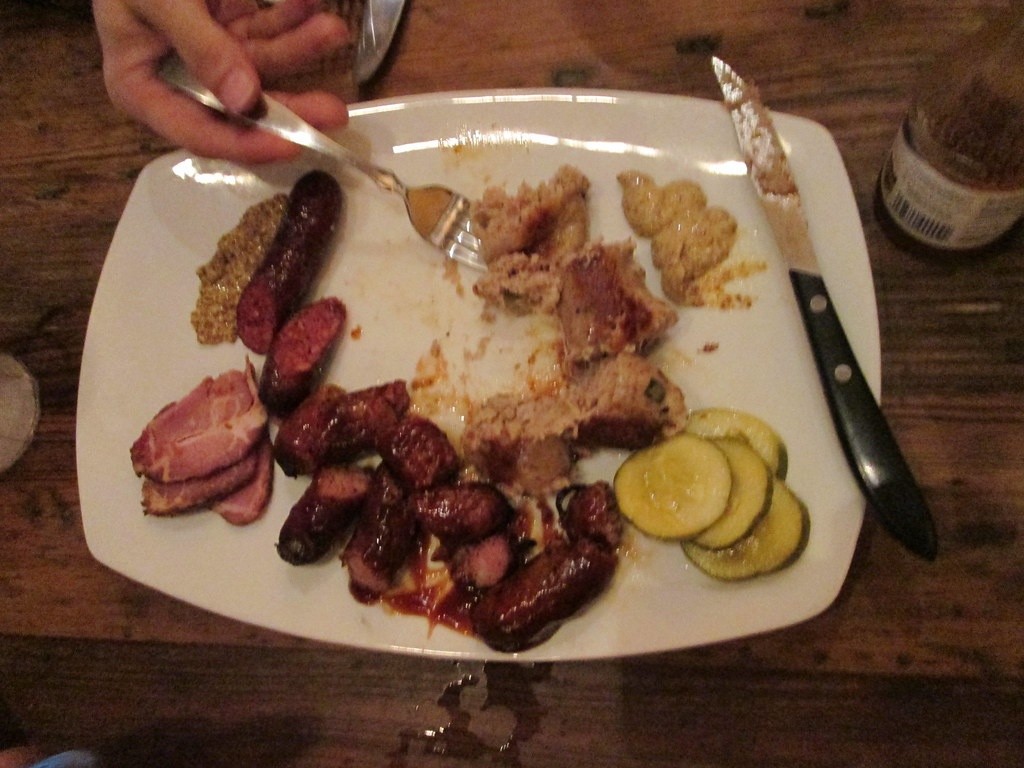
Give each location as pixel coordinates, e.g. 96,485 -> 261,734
612,406 -> 810,583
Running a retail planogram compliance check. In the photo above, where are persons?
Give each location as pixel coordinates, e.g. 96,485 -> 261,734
89,1 -> 352,163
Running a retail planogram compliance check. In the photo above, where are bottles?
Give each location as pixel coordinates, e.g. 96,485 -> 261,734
873,0 -> 1024,266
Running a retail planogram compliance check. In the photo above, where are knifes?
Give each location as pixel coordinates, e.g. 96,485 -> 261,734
712,55 -> 943,560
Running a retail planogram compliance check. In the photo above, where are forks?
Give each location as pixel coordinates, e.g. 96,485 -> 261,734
159,55 -> 488,270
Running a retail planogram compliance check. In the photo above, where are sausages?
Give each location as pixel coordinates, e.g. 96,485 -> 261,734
233,168 -> 624,653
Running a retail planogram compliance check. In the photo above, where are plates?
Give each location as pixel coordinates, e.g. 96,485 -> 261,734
76,86 -> 881,662
168,0 -> 405,88
